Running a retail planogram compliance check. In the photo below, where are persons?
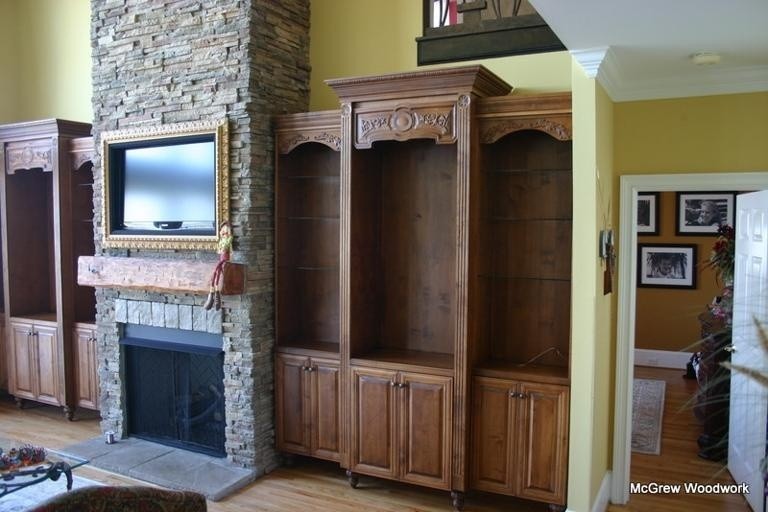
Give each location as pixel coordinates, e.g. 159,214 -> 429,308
204,221 -> 233,311
697,201 -> 721,226
654,257 -> 675,278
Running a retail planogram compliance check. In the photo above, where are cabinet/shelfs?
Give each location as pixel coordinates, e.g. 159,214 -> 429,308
270,63 -> 573,511
693,311 -> 732,459
2,118 -> 102,423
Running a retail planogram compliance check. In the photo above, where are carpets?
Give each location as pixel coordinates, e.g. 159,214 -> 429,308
0,463 -> 109,512
630,378 -> 667,455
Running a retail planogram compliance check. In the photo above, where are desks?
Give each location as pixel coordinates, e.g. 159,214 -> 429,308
0,438 -> 91,493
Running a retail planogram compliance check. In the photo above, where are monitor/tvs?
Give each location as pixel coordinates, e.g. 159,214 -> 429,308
109,131 -> 215,235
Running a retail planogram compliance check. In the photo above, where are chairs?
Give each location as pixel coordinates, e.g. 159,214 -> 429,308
27,483 -> 208,512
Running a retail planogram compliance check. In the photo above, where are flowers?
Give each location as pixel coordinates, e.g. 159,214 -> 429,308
698,222 -> 734,326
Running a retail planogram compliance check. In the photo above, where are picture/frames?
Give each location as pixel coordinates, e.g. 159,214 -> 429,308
637,243 -> 697,290
636,191 -> 660,236
676,191 -> 735,236
